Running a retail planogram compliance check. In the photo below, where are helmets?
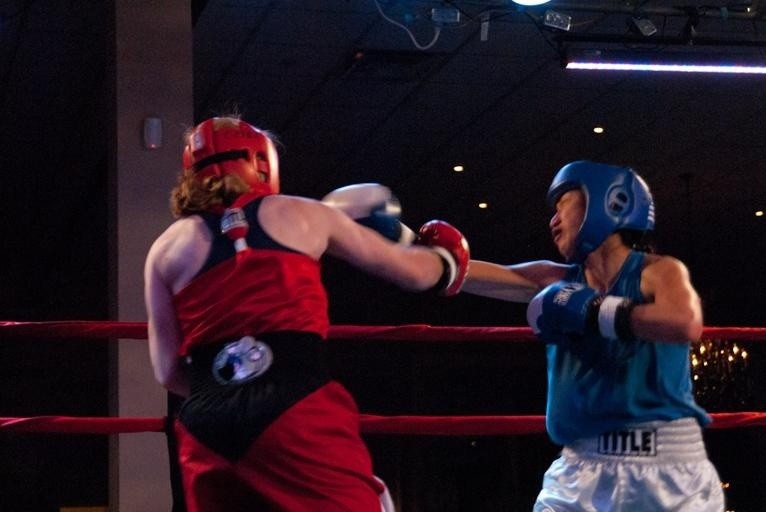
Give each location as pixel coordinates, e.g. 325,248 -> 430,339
544,160 -> 658,268
180,115 -> 284,213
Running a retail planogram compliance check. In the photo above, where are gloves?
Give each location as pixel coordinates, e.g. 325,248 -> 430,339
318,179 -> 415,254
524,275 -> 640,348
415,215 -> 476,300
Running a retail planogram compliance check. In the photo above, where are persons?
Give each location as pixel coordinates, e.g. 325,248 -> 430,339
144,116 -> 471,512
319,159 -> 726,511
